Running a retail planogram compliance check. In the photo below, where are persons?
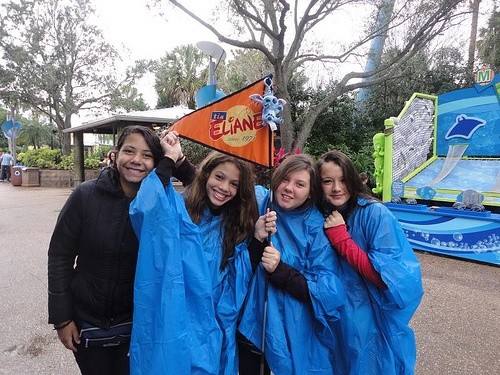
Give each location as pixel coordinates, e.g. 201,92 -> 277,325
49,124 -> 162,375
132,135 -> 278,375
163,130 -> 334,375
0,151 -> 14,182
314,150 -> 423,375
108,150 -> 116,165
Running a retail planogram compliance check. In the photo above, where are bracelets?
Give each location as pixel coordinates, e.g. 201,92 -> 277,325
177,155 -> 184,161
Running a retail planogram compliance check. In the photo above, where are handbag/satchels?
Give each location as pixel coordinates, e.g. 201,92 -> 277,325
71,318 -> 133,359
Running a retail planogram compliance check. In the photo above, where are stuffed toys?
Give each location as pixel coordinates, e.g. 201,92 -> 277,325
250,94 -> 285,126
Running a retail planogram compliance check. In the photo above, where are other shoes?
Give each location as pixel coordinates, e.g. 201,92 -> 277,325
0,179 -> 10,183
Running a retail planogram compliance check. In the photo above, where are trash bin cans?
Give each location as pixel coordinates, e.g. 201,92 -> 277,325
22,167 -> 39,187
11,166 -> 24,186
